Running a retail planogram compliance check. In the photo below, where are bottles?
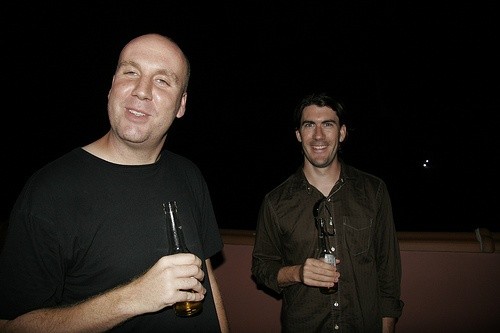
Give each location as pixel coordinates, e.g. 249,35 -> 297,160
161,201 -> 205,319
315,217 -> 339,295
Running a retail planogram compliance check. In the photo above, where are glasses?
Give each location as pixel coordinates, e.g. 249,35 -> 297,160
313,197 -> 335,236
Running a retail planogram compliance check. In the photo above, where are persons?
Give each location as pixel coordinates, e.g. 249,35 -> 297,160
0,33 -> 231,333
250,94 -> 404,333
